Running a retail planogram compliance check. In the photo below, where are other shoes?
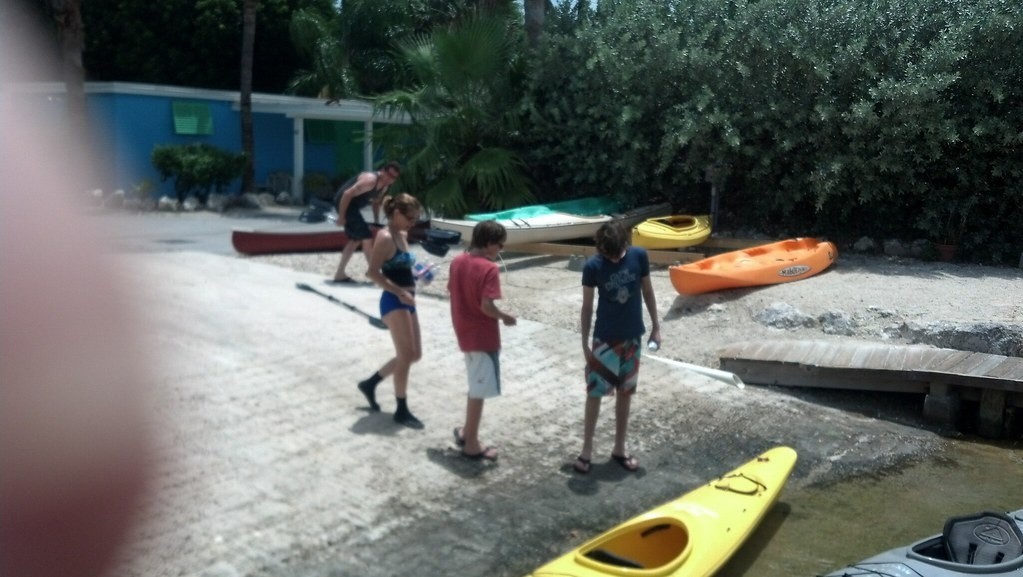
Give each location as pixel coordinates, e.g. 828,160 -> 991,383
358,379 -> 381,411
393,410 -> 424,430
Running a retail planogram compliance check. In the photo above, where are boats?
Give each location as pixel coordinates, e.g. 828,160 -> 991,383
464,193 -> 622,222
231,224 -> 386,256
803,504 -> 1023,577
669,235 -> 838,299
430,199 -> 675,248
631,213 -> 717,249
524,446 -> 800,577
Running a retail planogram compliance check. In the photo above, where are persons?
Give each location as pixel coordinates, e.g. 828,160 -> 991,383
357,194 -> 424,430
332,162 -> 401,284
447,221 -> 516,462
562,221 -> 661,475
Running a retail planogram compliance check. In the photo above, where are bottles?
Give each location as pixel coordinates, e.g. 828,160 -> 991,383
419,264 -> 440,285
327,215 -> 337,223
649,342 -> 657,351
412,256 -> 431,277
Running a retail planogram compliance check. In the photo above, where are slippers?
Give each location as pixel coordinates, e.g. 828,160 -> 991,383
333,277 -> 356,283
462,447 -> 498,462
454,426 -> 466,446
573,456 -> 592,474
611,451 -> 640,472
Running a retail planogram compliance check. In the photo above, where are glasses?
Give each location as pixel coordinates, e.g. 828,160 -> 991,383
387,170 -> 399,181
497,242 -> 504,249
401,212 -> 419,223
